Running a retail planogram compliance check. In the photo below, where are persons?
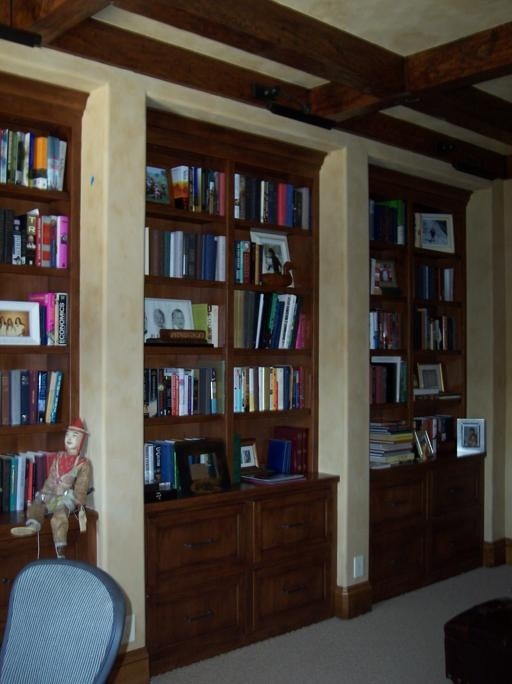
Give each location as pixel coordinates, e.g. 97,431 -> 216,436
9,415 -> 92,559
0,316 -> 25,337
465,427 -> 475,447
468,433 -> 477,447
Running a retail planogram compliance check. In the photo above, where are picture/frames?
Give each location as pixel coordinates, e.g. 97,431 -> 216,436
418,362 -> 447,394
415,212 -> 458,254
0,299 -> 40,347
456,418 -> 485,457
145,295 -> 198,341
239,437 -> 262,472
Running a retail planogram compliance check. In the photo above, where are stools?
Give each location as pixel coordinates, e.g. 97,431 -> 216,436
444,598 -> 512,684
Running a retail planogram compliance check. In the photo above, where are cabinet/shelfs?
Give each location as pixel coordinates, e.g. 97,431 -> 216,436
368,161 -> 486,606
144,102 -> 368,680
0,74 -> 97,638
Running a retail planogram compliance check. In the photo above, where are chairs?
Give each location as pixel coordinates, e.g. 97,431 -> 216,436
0,560 -> 131,684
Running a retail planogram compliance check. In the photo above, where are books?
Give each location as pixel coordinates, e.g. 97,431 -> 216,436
234,174 -> 311,230
234,365 -> 304,412
144,437 -> 215,490
2,369 -> 61,425
266,426 -> 309,474
1,130 -> 68,191
145,228 -> 226,281
144,367 -> 219,416
232,240 -> 263,284
145,304 -> 219,347
1,209 -> 68,270
369,201 -> 453,470
234,290 -> 305,350
146,166 -> 225,217
0,450 -> 62,513
29,292 -> 66,346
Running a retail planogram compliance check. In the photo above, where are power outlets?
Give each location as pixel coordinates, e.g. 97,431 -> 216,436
353,557 -> 365,577
